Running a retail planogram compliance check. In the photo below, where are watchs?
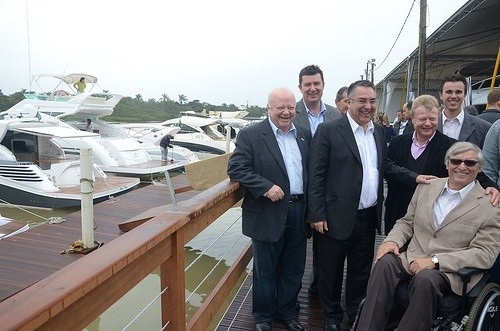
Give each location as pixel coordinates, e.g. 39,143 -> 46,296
431,254 -> 439,270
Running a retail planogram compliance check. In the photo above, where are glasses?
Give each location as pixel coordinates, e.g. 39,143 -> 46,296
350,97 -> 377,104
450,158 -> 478,166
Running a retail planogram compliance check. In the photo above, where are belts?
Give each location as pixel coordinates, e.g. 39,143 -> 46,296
289,195 -> 306,203
358,208 -> 371,214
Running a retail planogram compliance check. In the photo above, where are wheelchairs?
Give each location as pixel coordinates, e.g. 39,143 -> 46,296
350,235 -> 500,331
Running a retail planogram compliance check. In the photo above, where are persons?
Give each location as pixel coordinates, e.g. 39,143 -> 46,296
160,134 -> 174,161
313,80 -> 438,331
84,119 -> 94,133
73,77 -> 86,95
357,141 -> 500,331
291,64 -> 342,316
375,74 -> 500,252
335,87 -> 350,114
228,87 -> 312,331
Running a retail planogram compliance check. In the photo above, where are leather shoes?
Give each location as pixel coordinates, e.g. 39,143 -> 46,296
349,316 -> 356,325
256,322 -> 272,331
285,319 -> 303,331
325,323 -> 338,331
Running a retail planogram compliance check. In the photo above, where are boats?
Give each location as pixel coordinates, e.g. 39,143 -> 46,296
0,73 -> 258,208
